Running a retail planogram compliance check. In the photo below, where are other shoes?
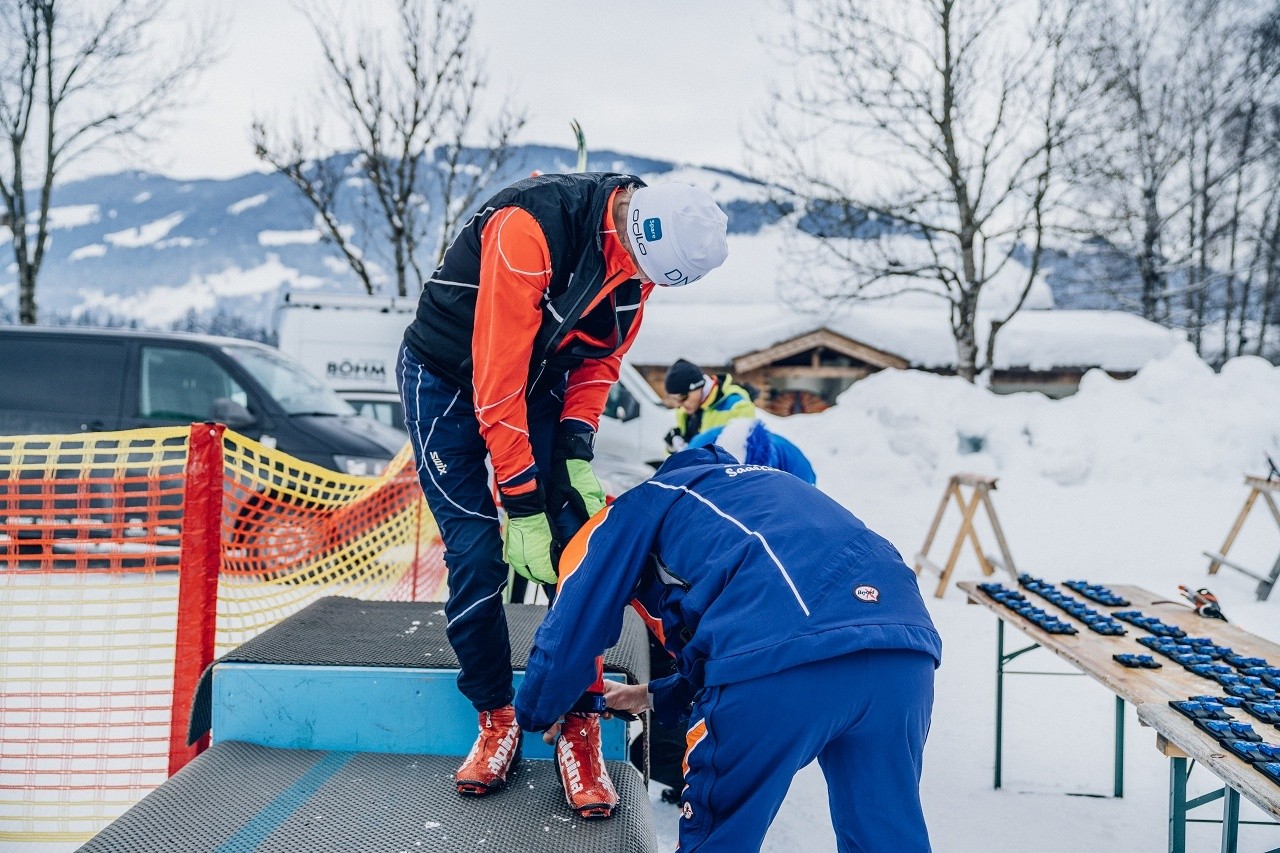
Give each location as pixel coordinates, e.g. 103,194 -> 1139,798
455,704 -> 523,796
554,714 -> 616,819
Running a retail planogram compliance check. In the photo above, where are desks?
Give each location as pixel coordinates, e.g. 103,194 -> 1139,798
953,579 -> 1279,853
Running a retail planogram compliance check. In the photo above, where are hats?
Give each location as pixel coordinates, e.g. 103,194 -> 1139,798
664,360 -> 705,395
627,180 -> 729,287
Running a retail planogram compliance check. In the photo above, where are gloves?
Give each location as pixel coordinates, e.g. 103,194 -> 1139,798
500,490 -> 561,585
563,457 -> 607,523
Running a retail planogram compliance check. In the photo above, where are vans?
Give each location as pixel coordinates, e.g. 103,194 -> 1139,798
0,325 -> 422,584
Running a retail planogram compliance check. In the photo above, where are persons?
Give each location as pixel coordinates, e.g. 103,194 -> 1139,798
681,419 -> 816,489
515,443 -> 943,853
396,172 -> 728,818
664,358 -> 755,457
